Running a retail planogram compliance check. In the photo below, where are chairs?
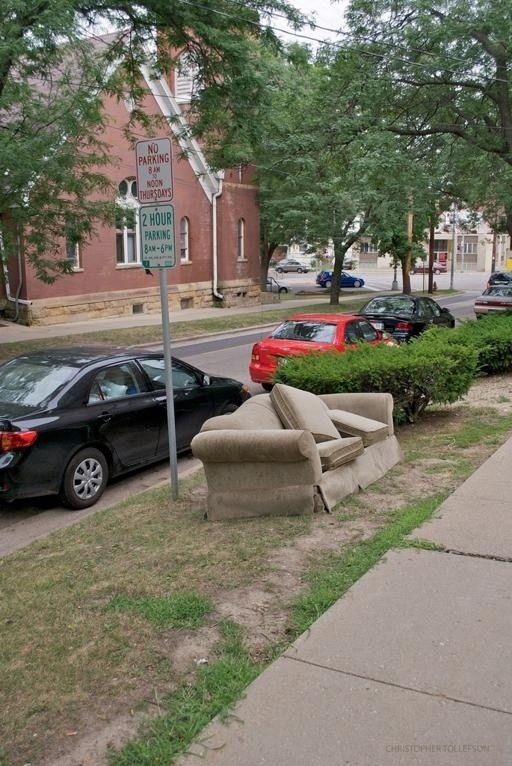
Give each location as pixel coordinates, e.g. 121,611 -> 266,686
102,370 -> 130,398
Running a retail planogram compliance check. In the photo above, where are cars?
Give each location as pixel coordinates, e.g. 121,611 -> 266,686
473,272 -> 512,320
359,295 -> 455,344
271,259 -> 309,273
249,313 -> 400,390
0,348 -> 252,510
316,271 -> 364,288
409,262 -> 447,275
266,277 -> 288,293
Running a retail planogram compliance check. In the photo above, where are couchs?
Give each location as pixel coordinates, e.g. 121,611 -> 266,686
191,382 -> 404,521
